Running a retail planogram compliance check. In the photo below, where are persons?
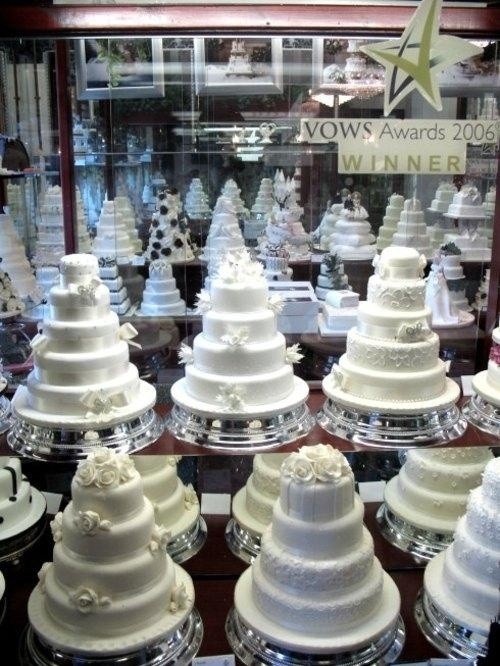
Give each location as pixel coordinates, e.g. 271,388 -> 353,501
122,50 -> 137,76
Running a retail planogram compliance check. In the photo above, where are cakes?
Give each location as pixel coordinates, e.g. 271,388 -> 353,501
72,120 -> 89,159
0,168 -> 497,337
27,444 -> 195,655
423,456 -> 500,637
331,244 -> 451,402
126,453 -> 200,543
486,326 -> 500,392
477,98 -> 499,120
26,253 -> 140,416
179,257 -> 304,413
345,39 -> 367,73
232,452 -> 294,535
0,457 -> 33,532
383,446 -> 496,535
234,443 -> 401,654
226,41 -> 252,72
